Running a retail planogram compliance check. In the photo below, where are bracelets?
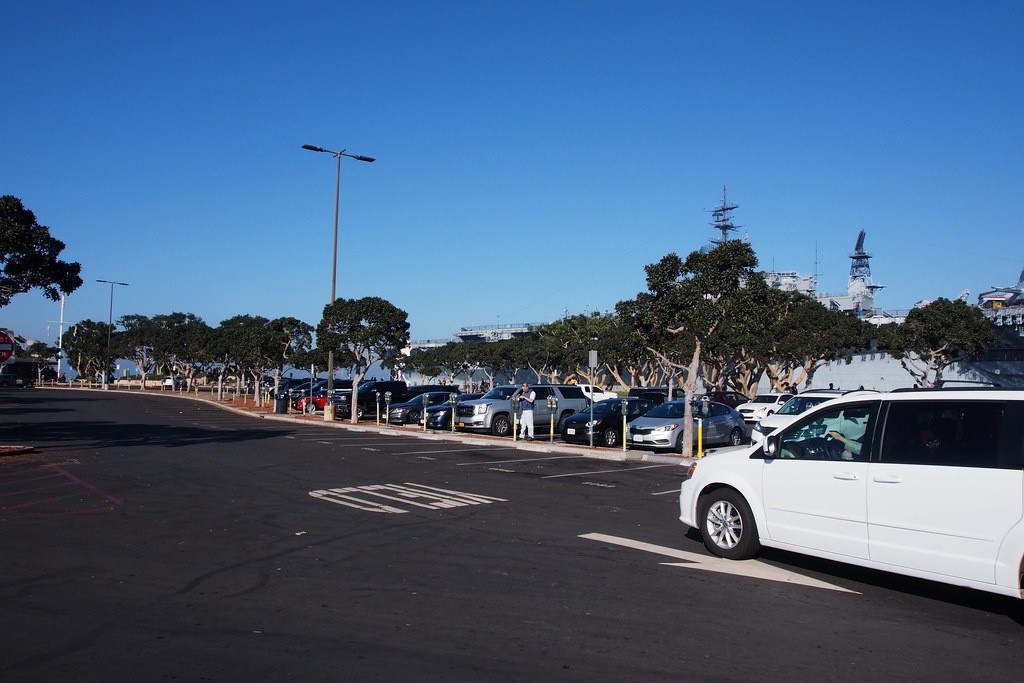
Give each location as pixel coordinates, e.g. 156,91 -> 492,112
523,396 -> 525,399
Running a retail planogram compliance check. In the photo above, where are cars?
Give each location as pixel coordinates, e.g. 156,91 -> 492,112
269,376 -> 487,444
0,359 -> 40,389
624,380 -> 1024,600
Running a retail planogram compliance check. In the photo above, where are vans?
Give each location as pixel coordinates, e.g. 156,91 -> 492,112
454,385 -> 588,436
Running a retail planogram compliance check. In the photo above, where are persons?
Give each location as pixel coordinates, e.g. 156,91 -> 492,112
828,430 -> 862,460
770,382 -> 798,395
606,383 -> 613,391
517,382 -> 536,440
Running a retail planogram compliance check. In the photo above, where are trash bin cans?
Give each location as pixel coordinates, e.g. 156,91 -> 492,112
274,392 -> 288,414
324,401 -> 335,421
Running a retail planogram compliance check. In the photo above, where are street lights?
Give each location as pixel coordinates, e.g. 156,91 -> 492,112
303,144 -> 377,308
96,278 -> 129,383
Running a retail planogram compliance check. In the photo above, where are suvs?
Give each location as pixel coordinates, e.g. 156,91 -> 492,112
576,385 -> 619,402
561,397 -> 658,448
161,374 -> 187,388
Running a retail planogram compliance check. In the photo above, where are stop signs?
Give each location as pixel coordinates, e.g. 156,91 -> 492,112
0,331 -> 14,362
